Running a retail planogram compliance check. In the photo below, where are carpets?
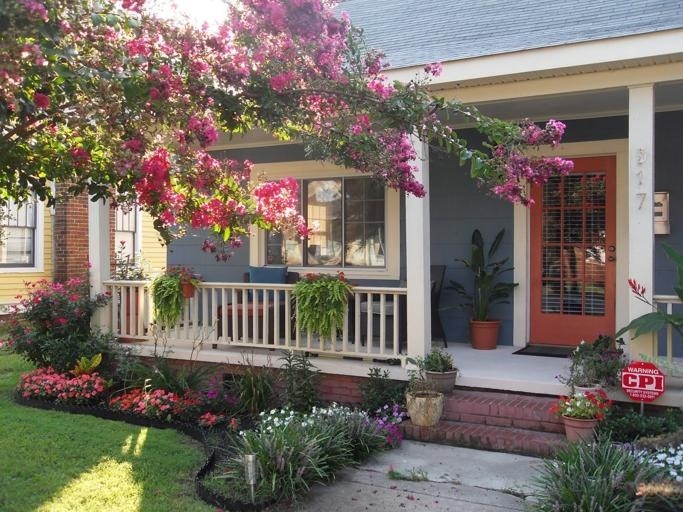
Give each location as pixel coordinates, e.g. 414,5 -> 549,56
511,341 -> 579,358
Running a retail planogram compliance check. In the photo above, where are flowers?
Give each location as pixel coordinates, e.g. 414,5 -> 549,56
556,334 -> 627,385
178,267 -> 202,286
547,387 -> 614,419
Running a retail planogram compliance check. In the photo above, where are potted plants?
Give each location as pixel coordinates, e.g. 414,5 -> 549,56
404,344 -> 458,428
442,226 -> 519,351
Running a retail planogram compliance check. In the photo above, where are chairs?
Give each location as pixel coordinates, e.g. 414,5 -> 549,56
210,272 -> 299,352
348,265 -> 447,356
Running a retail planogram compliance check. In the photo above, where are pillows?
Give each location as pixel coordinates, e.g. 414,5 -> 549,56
246,266 -> 289,302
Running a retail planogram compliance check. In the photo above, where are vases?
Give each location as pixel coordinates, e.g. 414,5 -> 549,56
180,281 -> 194,298
573,383 -> 606,396
561,413 -> 605,444
